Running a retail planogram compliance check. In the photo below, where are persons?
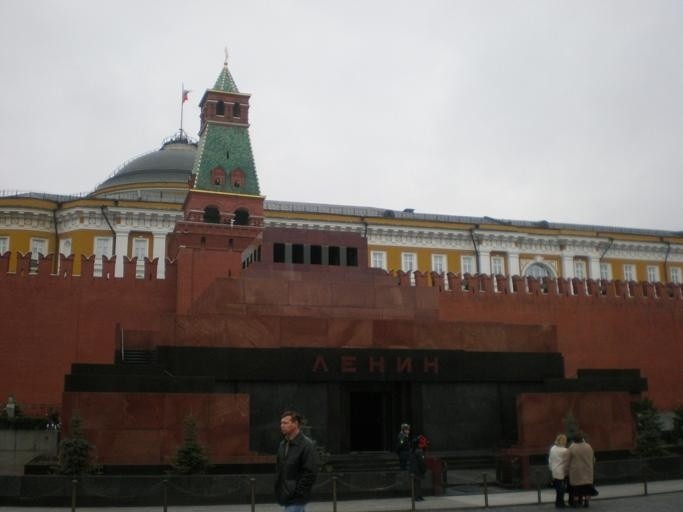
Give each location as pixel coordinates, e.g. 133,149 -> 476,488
407,433 -> 429,501
568,432 -> 597,508
273,409 -> 319,512
396,421 -> 415,471
546,435 -> 569,509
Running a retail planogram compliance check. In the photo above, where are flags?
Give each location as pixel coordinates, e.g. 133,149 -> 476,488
182,89 -> 190,103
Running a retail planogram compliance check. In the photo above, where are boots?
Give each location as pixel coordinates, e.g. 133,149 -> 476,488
555,499 -> 589,508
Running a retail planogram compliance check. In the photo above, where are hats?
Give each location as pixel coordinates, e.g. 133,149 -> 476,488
400,423 -> 410,430
